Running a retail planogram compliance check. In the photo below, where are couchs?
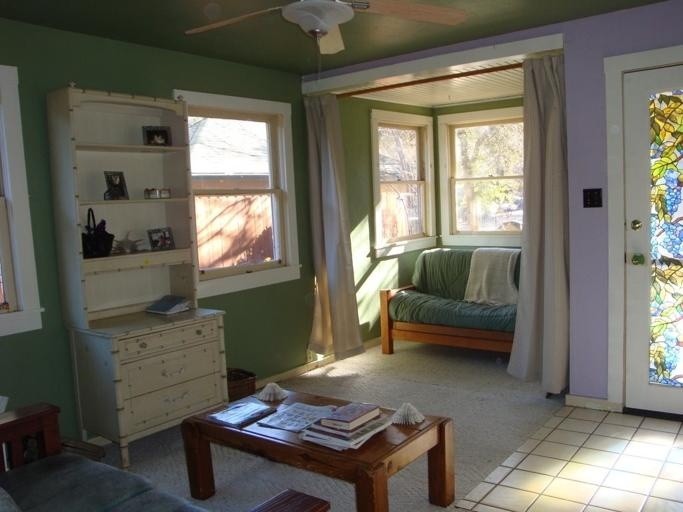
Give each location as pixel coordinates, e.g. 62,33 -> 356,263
0,400 -> 332,512
379,247 -> 526,354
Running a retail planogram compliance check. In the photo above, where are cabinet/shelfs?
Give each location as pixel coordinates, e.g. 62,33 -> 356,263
51,91 -> 230,470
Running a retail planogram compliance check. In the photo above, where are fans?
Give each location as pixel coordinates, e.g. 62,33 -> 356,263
184,0 -> 468,56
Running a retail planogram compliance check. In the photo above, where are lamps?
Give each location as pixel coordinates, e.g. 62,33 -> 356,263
281,0 -> 354,42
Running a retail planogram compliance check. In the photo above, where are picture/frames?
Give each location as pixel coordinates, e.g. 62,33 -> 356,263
0,64 -> 44,337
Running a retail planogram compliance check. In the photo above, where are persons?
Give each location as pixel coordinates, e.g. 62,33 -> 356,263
147,227 -> 175,253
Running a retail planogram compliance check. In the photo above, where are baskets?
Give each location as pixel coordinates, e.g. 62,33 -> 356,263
226,369 -> 256,400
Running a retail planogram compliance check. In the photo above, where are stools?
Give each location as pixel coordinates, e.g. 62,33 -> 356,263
225,367 -> 260,400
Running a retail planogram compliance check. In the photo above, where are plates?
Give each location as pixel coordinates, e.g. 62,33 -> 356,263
143,188 -> 171,199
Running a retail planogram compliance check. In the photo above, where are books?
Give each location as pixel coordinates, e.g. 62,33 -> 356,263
145,294 -> 189,315
209,396 -> 276,429
299,403 -> 394,450
258,401 -> 337,431
